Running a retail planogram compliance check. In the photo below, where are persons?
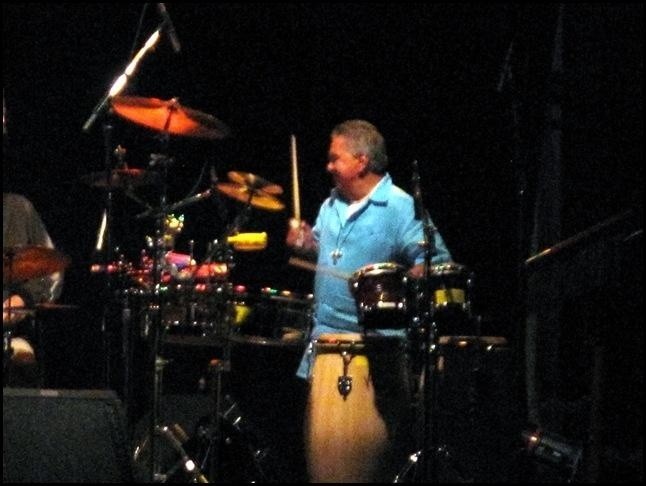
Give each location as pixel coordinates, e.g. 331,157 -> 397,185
287,120 -> 452,381
0,193 -> 64,325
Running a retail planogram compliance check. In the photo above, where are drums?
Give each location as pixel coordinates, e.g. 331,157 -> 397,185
347,258 -> 413,325
229,280 -> 314,347
304,326 -> 397,485
157,282 -> 232,345
118,265 -> 156,314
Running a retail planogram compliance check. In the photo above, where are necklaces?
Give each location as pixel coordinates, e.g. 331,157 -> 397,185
330,217 -> 357,265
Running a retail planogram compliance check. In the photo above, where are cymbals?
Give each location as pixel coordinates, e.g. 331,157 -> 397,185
217,182 -> 288,209
80,168 -> 156,193
6,297 -> 80,316
2,242 -> 72,286
418,262 -> 486,323
226,171 -> 281,193
110,97 -> 226,140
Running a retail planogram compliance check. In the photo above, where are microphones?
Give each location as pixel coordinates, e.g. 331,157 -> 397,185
411,160 -> 427,222
157,2 -> 181,54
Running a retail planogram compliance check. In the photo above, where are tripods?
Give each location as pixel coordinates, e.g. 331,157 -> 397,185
192,277 -> 265,481
129,218 -> 206,480
394,281 -> 473,481
